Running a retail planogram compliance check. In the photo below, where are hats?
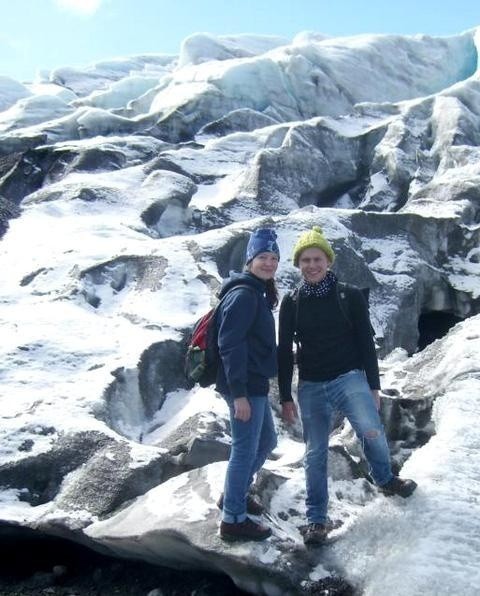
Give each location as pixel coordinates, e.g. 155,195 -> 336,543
244,226 -> 282,266
291,225 -> 337,269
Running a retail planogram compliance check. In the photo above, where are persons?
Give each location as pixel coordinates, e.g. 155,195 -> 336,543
212,228 -> 282,543
278,224 -> 420,544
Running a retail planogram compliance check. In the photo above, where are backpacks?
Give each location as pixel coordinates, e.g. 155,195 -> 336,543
181,282 -> 261,390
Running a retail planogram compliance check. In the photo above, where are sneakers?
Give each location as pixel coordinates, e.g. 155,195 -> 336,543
215,490 -> 266,517
302,522 -> 329,545
219,516 -> 274,543
379,473 -> 418,499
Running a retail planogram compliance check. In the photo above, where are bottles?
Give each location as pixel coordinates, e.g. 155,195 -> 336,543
188,362 -> 206,382
